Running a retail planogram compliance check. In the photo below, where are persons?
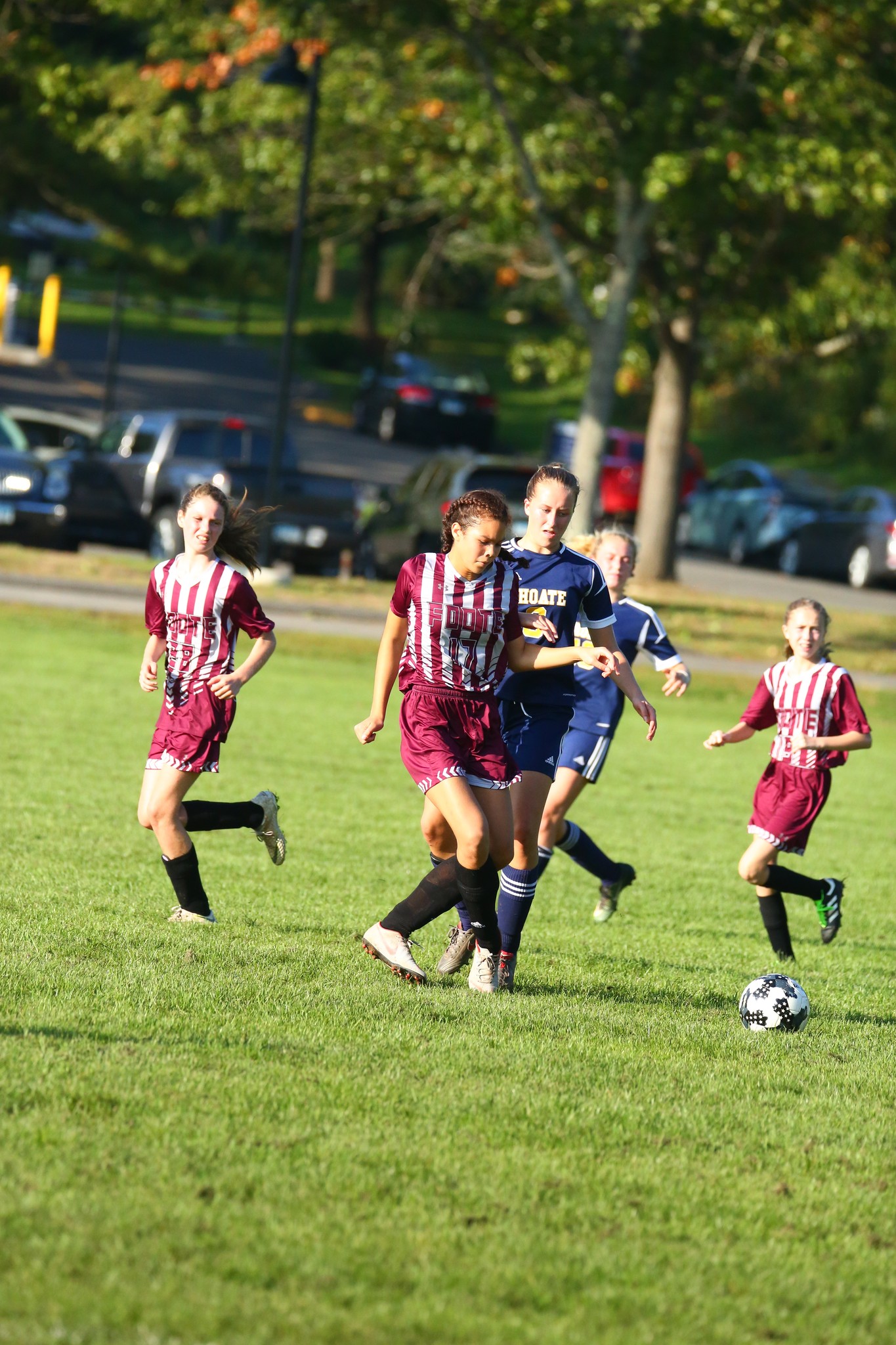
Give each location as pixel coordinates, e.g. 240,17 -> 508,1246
354,489 -> 620,993
138,482 -> 287,924
702,596 -> 872,964
420,461 -> 657,991
535,520 -> 692,922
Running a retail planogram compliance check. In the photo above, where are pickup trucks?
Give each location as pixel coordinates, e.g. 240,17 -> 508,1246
89,406 -> 388,577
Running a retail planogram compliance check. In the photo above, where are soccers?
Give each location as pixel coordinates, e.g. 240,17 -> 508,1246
738,973 -> 811,1033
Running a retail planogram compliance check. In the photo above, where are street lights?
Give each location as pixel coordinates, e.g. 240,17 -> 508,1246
247,52 -> 323,581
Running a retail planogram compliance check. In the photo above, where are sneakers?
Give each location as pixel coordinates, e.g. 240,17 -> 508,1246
167,906 -> 217,922
437,921 -> 476,975
814,878 -> 844,944
251,790 -> 286,865
593,863 -> 635,924
498,952 -> 517,993
362,921 -> 427,986
469,932 -> 502,993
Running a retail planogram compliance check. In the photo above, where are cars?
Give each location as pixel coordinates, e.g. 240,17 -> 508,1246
351,350 -> 496,450
0,399 -> 148,554
767,482 -> 896,594
674,459 -> 799,566
355,447 -> 549,584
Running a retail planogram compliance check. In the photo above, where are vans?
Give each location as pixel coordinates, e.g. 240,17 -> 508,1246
550,418 -> 703,524
